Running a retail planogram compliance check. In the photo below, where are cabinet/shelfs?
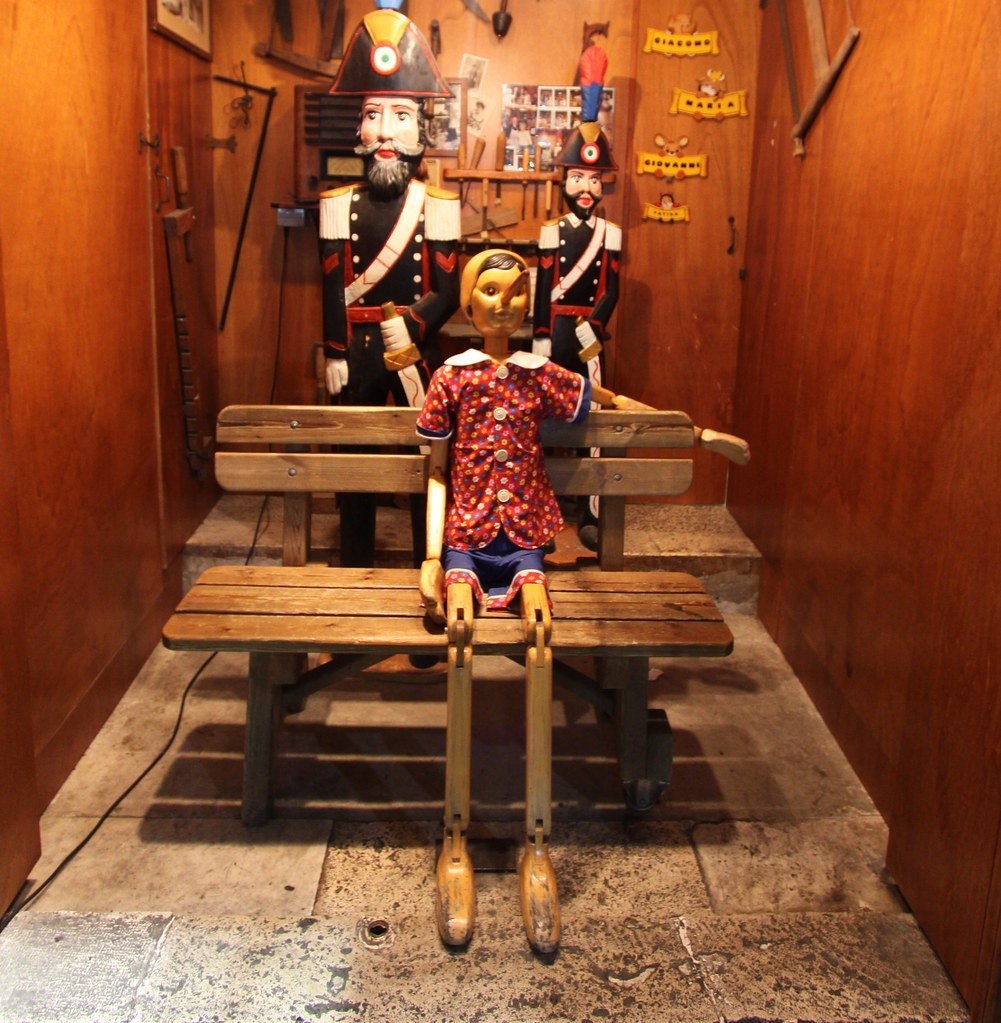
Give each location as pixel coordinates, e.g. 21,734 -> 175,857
443,169 -> 617,251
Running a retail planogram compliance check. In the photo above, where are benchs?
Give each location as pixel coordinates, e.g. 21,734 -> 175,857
160,404 -> 739,821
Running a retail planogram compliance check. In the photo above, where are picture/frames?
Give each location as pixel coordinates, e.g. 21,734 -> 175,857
147,0 -> 216,63
424,77 -> 468,158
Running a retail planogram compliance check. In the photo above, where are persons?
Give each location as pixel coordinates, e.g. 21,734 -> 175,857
467,102 -> 485,130
532,46 -> 621,554
466,64 -> 479,89
500,85 -> 613,172
319,0 -> 461,669
415,249 -> 751,954
